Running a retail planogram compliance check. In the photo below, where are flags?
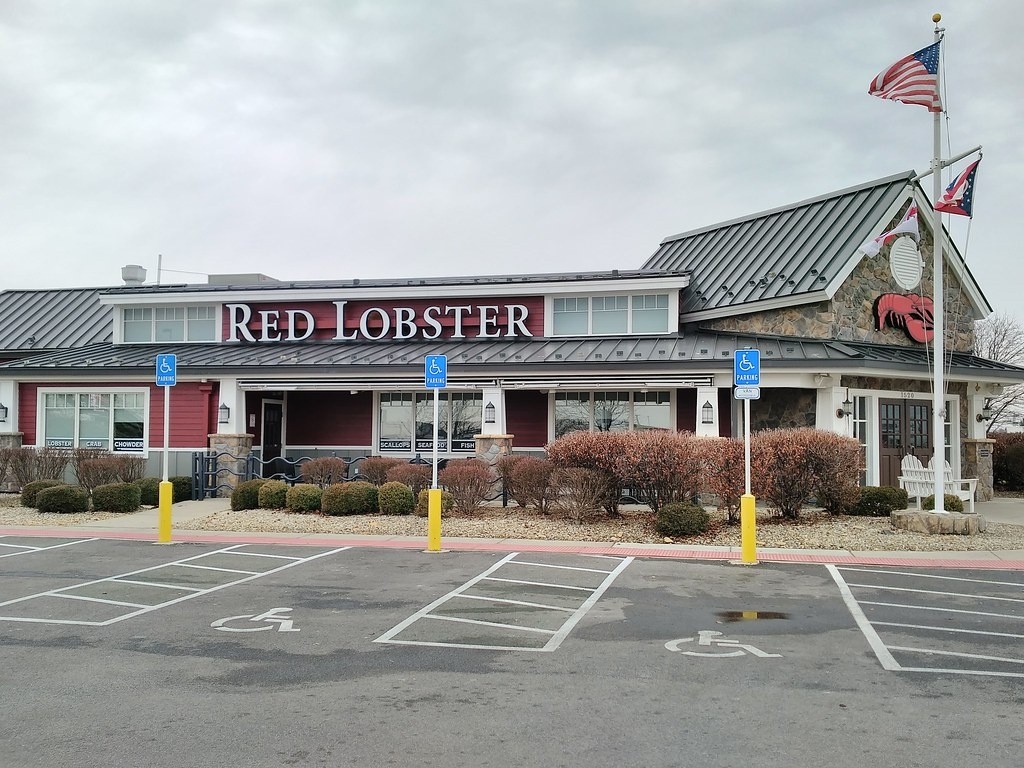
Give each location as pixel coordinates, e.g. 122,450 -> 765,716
856,197 -> 921,259
932,158 -> 982,218
868,39 -> 944,112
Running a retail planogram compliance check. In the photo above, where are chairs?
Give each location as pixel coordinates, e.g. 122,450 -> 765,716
928,456 -> 979,514
897,454 -> 935,510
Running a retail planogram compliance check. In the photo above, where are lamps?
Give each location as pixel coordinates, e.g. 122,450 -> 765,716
702,400 -> 714,424
982,398 -> 993,421
485,401 -> 495,423
219,403 -> 230,424
842,388 -> 853,417
0,403 -> 8,422
